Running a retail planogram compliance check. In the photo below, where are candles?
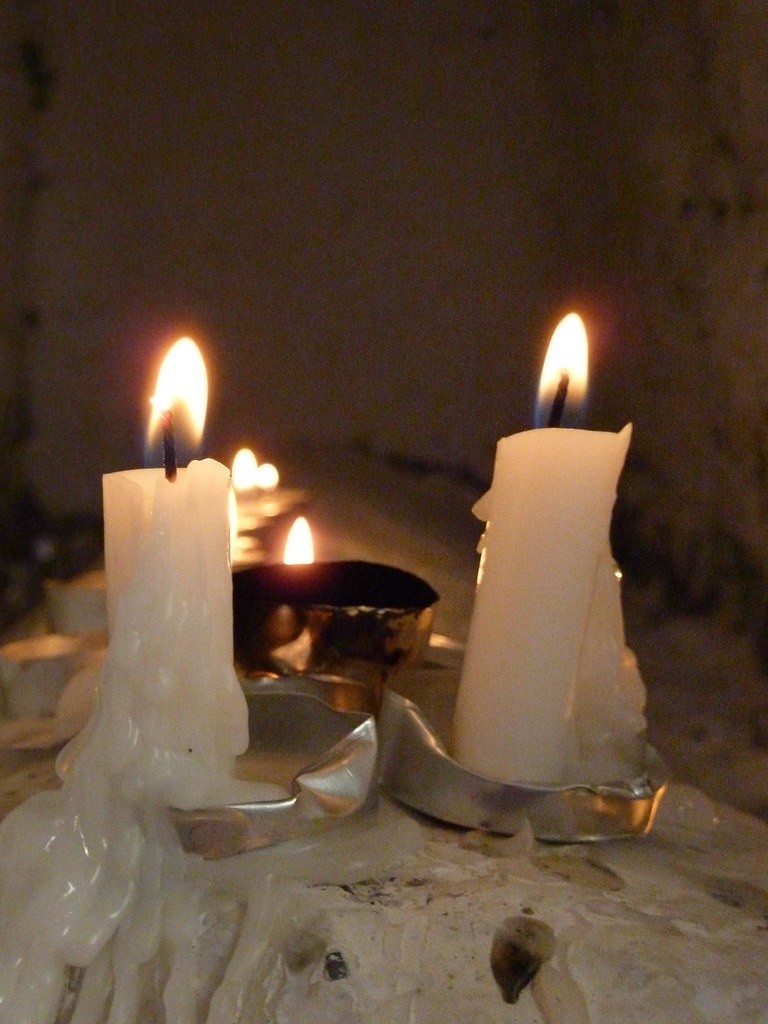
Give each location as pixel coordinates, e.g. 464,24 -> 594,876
102,336 -> 249,758
451,312 -> 647,786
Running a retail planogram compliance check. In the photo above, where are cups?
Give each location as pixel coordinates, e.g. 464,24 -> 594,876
0,631 -> 81,717
42,570 -> 109,633
